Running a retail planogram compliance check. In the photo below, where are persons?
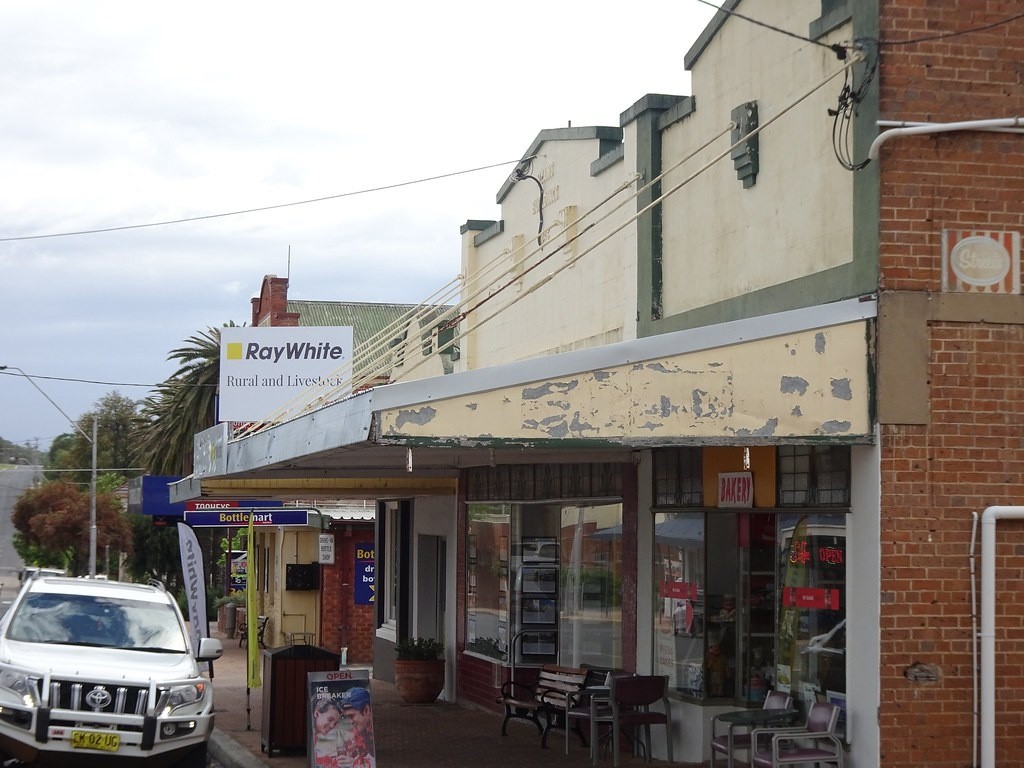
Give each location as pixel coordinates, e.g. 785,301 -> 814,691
704,594 -> 737,697
311,687 -> 374,768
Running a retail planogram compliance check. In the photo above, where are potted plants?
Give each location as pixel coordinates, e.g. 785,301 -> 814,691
394,638 -> 444,705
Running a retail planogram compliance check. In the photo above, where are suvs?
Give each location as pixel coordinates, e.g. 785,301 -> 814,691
0,571 -> 224,768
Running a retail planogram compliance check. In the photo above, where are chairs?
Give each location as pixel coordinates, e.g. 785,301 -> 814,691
590,674 -> 672,768
751,703 -> 844,768
565,667 -> 623,755
712,690 -> 793,768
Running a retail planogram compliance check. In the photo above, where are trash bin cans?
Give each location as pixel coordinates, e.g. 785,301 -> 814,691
259,644 -> 342,758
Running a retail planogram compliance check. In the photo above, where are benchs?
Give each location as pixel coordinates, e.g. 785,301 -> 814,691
497,664 -> 594,750
237,616 -> 266,651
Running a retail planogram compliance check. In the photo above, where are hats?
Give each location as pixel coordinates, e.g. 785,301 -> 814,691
340,686 -> 370,709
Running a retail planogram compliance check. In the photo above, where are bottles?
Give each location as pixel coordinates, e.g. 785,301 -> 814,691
336,731 -> 347,757
341,731 -> 359,759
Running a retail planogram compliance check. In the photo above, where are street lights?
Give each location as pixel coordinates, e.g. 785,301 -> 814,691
0,364 -> 100,578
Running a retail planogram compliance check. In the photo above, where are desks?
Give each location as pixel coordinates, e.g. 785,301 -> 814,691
718,708 -> 799,752
